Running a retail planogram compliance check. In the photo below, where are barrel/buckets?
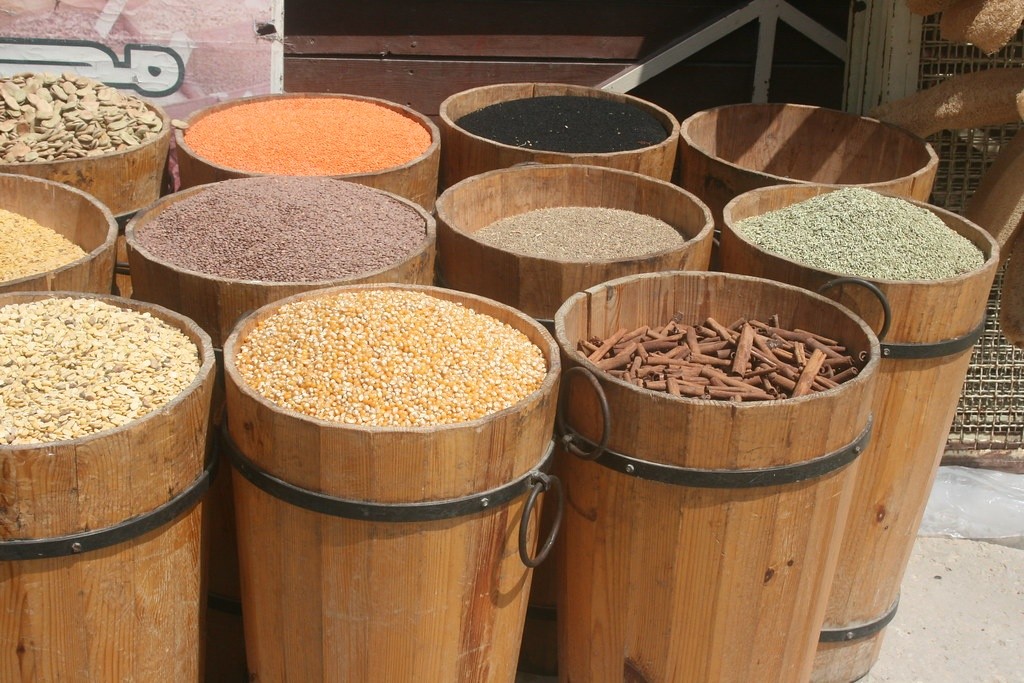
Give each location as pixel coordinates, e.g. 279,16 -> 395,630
682,102 -> 938,270
0,96 -> 171,301
552,269 -> 881,683
0,290 -> 218,683
0,171 -> 119,294
174,92 -> 442,213
125,181 -> 437,683
439,82 -> 681,193
435,163 -> 716,678
224,284 -> 564,683
722,185 -> 1001,683
